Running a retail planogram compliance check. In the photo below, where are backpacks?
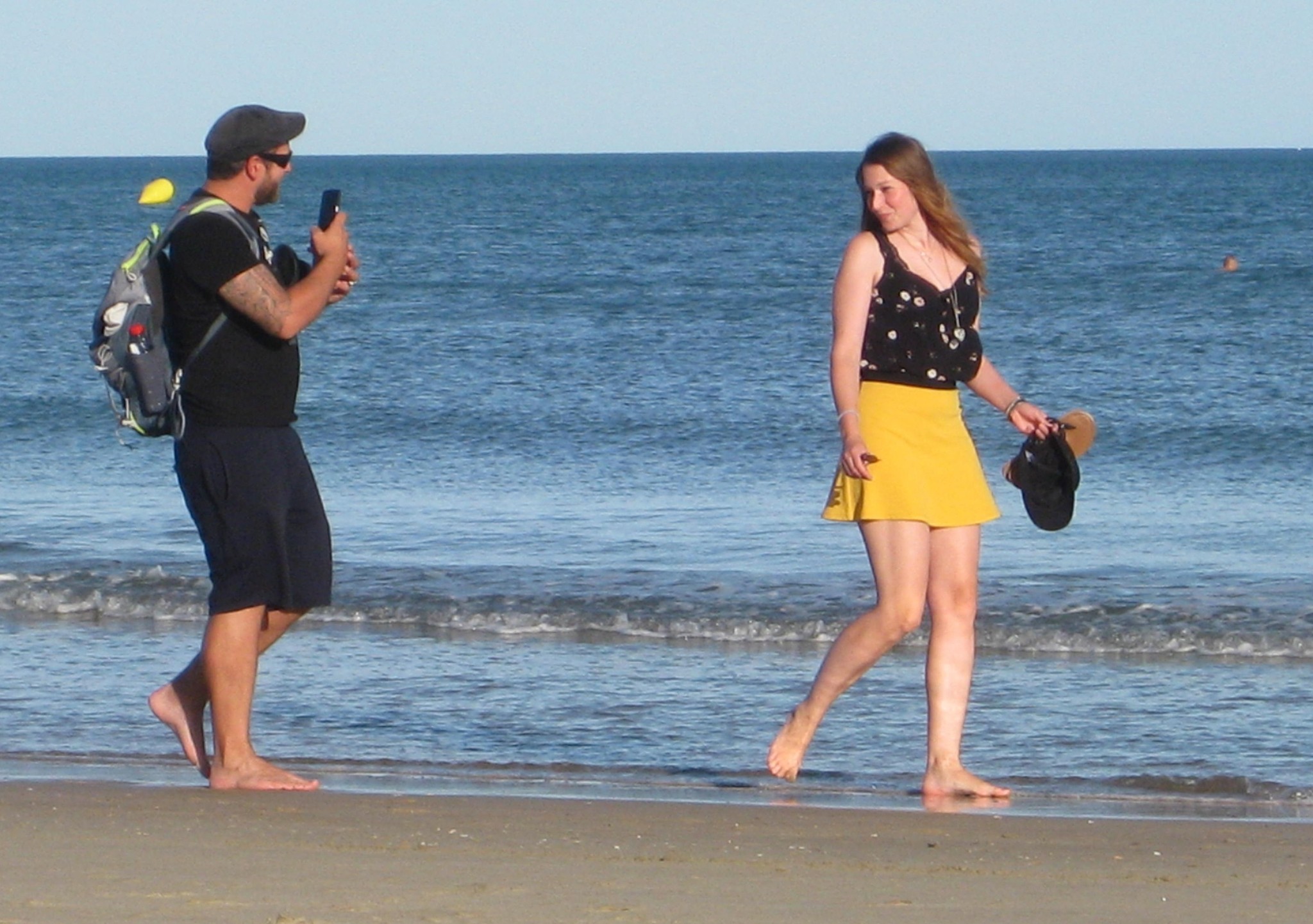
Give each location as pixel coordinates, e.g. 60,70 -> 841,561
90,197 -> 260,437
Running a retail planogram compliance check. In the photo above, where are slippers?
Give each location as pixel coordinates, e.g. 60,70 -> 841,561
1001,410 -> 1096,487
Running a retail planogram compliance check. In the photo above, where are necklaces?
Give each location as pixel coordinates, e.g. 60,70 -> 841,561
897,227 -> 966,342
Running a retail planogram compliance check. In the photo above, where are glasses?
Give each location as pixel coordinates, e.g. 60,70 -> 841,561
258,151 -> 295,169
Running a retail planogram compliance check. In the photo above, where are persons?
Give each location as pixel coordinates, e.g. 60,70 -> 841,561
766,133 -> 1057,799
152,105 -> 358,788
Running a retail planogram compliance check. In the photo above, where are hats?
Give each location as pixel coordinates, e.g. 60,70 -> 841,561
205,103 -> 306,159
1013,428 -> 1080,531
271,240 -> 311,288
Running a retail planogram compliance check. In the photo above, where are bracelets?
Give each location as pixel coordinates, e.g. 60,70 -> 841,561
837,410 -> 859,425
1006,396 -> 1026,422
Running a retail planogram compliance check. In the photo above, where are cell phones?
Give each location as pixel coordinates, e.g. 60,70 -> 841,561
319,190 -> 342,232
